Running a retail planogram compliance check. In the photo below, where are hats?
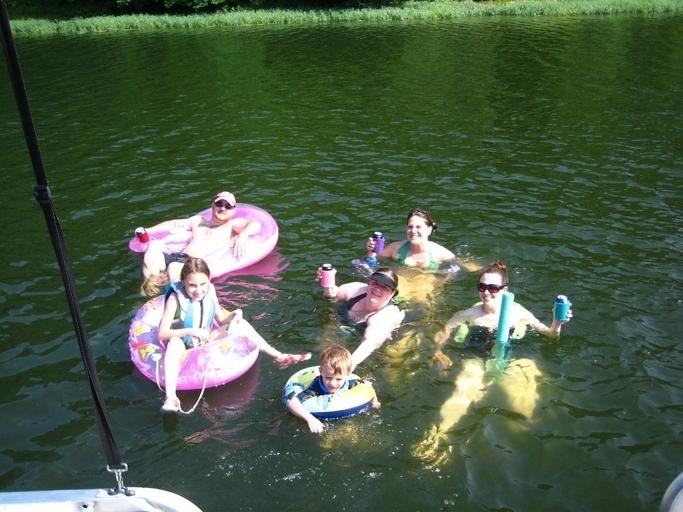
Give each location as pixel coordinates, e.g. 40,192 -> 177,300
213,191 -> 236,207
367,273 -> 396,292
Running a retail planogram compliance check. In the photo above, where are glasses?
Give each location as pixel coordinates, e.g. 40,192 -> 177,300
215,200 -> 232,209
477,282 -> 504,294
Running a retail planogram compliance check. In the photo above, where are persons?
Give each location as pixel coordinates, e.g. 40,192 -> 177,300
159,257 -> 312,415
133,190 -> 259,289
316,262 -> 406,372
413,264 -> 572,457
285,344 -> 381,449
367,208 -> 483,314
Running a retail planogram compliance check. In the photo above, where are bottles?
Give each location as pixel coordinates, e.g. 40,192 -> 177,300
135,227 -> 149,244
553,294 -> 569,321
453,324 -> 469,343
371,231 -> 384,254
320,263 -> 333,288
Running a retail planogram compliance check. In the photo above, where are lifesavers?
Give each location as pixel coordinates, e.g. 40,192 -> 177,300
281,366 -> 374,418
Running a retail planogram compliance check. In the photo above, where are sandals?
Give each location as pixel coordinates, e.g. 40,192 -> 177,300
162,397 -> 180,412
274,354 -> 305,369
